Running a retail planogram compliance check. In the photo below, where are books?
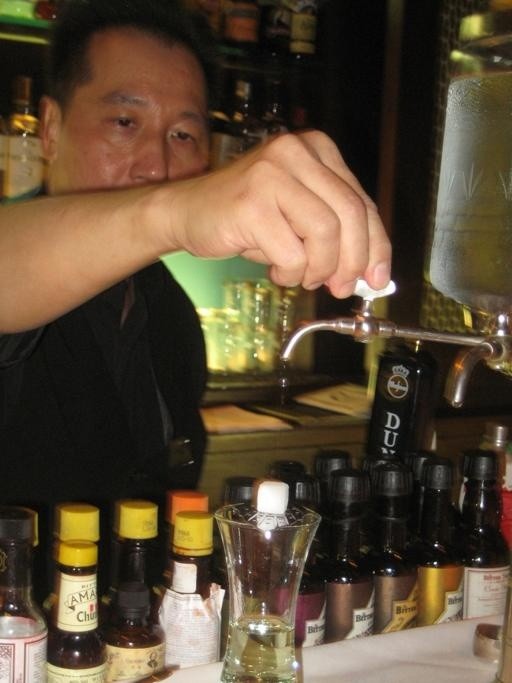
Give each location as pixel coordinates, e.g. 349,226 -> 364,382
201,399 -> 292,433
294,380 -> 372,420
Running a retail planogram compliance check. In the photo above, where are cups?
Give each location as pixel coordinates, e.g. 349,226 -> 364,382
195,277 -> 285,377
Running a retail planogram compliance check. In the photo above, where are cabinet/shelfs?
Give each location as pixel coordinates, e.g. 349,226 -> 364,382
192,395 -> 512,511
0,1 -> 407,402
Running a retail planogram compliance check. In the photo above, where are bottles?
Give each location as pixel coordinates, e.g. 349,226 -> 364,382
0,419 -> 512,681
1,0 -> 325,199
363,324 -> 422,458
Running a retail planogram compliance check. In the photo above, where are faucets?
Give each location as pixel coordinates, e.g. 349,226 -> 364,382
279,278 -> 486,363
443,332 -> 512,410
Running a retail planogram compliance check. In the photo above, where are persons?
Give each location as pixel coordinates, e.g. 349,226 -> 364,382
0,4 -> 396,598
